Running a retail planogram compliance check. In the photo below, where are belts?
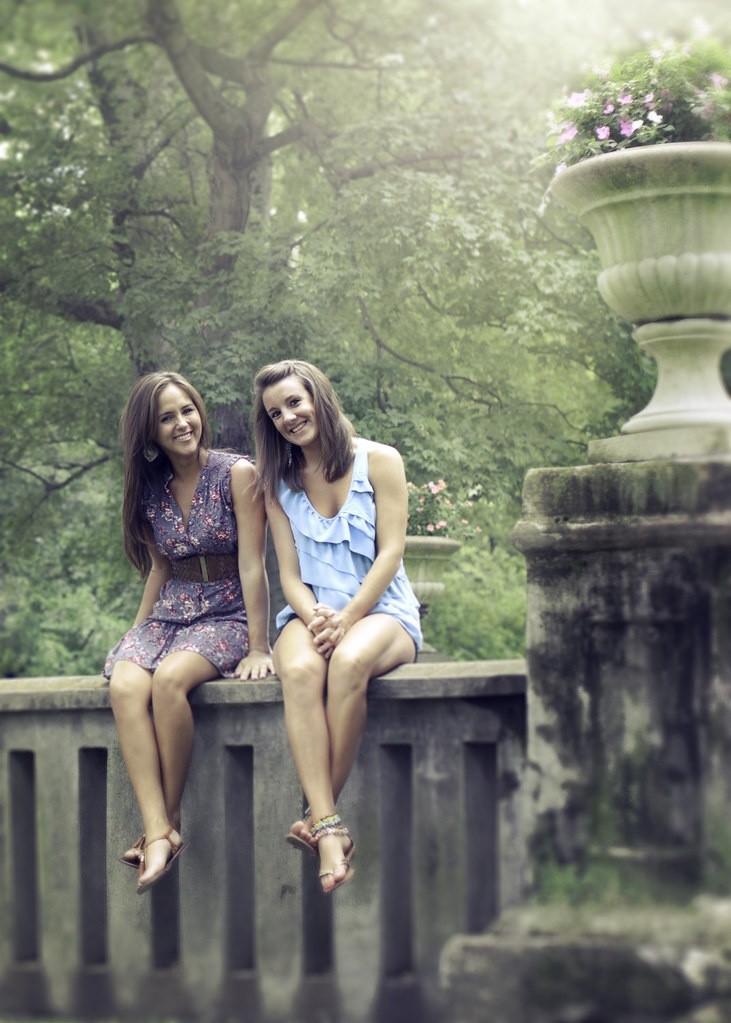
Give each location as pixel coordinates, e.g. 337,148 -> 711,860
171,552 -> 238,583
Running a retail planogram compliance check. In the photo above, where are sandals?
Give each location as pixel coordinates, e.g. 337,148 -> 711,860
135,827 -> 187,895
120,833 -> 145,869
310,814 -> 351,893
285,805 -> 319,857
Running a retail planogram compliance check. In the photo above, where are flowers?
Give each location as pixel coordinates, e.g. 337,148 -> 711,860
543,32 -> 731,175
405,480 -> 483,544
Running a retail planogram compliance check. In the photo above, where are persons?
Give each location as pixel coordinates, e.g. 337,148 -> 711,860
103,372 -> 275,895
254,359 -> 424,895
